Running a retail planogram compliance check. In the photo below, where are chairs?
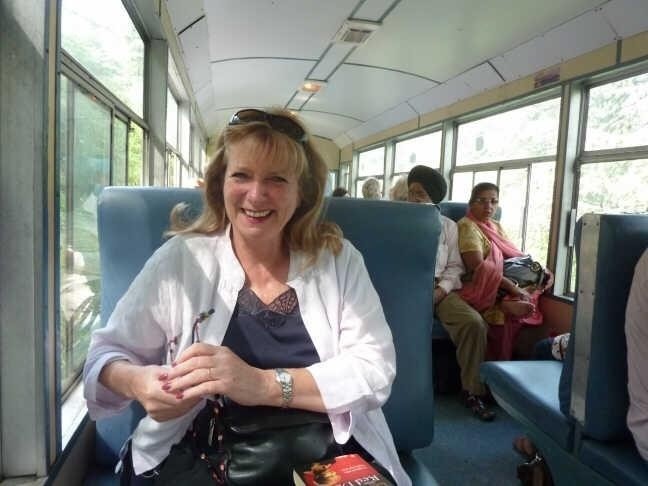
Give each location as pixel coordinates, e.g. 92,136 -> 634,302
82,184 -> 440,482
475,210 -> 647,485
436,201 -> 504,340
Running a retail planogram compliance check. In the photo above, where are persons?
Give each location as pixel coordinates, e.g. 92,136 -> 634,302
512,332 -> 571,460
625,247 -> 648,467
456,183 -> 550,361
361,177 -> 382,200
407,165 -> 496,421
388,174 -> 409,202
82,107 -> 412,486
332,188 -> 348,197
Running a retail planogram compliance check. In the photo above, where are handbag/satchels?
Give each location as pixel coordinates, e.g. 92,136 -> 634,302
503,255 -> 550,290
219,414 -> 336,484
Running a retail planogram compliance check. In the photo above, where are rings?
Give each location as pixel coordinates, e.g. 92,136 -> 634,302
208,367 -> 211,381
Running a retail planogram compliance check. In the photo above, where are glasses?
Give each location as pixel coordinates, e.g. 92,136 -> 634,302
473,196 -> 499,204
229,109 -> 308,145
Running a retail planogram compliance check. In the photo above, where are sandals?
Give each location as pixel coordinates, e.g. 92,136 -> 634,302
512,435 -> 534,458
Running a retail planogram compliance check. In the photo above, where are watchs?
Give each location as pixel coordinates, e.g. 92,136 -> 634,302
275,368 -> 294,409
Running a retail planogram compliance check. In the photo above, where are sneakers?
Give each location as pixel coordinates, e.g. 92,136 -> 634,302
465,394 -> 495,421
